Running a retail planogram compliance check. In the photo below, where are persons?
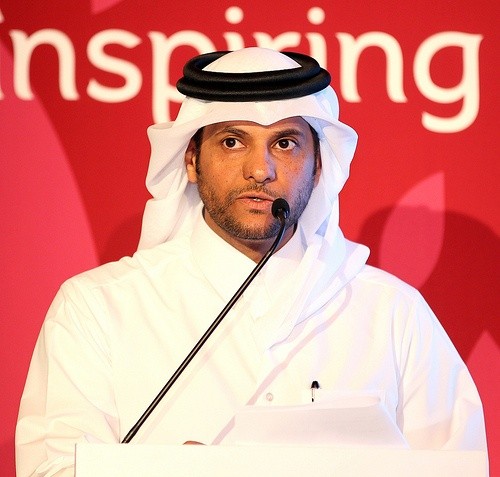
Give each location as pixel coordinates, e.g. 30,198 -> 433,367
15,47 -> 489,477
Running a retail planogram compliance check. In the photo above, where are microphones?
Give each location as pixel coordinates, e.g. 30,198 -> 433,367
121,199 -> 290,443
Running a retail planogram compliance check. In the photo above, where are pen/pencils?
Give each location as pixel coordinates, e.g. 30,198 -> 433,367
311,381 -> 319,402
